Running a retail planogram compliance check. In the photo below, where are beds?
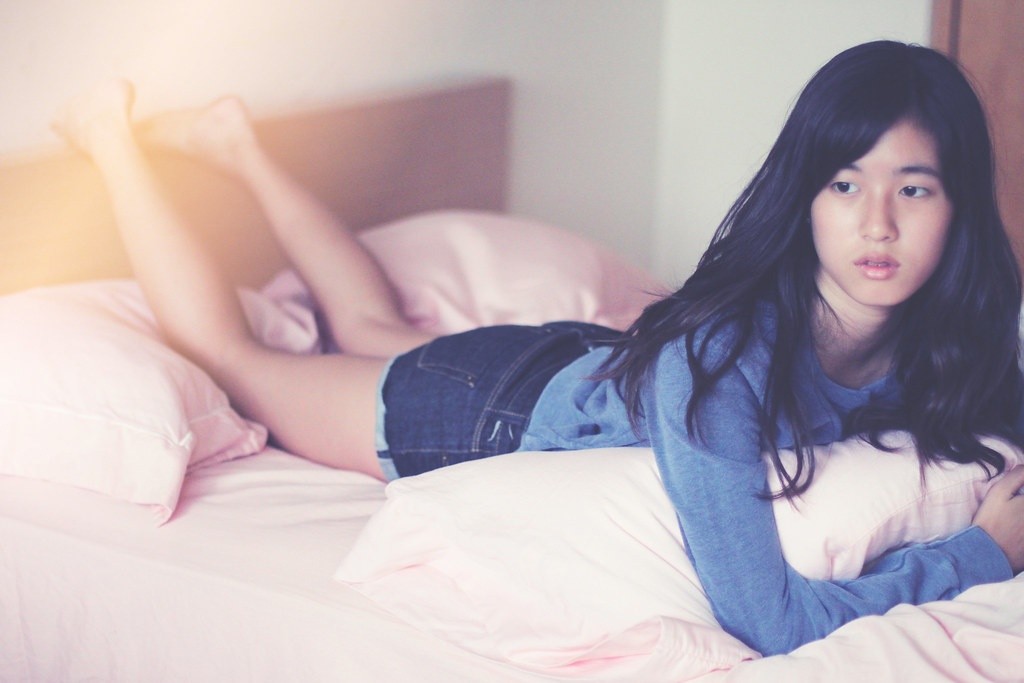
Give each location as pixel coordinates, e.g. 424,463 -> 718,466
6,207 -> 1024,680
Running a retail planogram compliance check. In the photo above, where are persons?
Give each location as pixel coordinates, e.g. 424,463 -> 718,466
41,34 -> 1024,665
